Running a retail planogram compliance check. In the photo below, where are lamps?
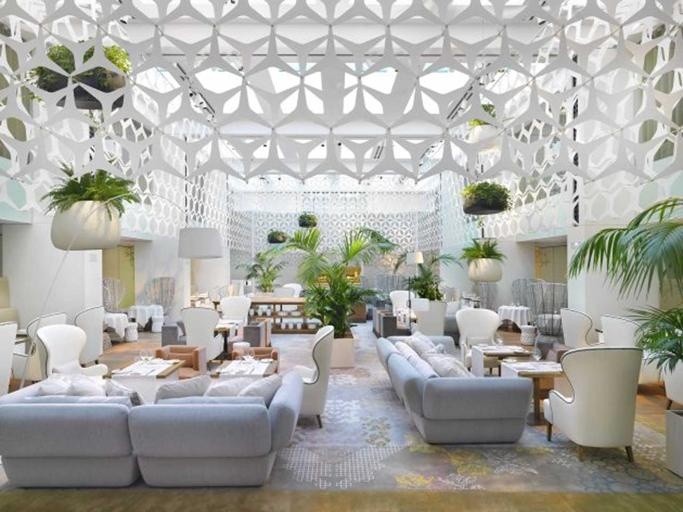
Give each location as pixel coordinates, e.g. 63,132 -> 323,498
385,245 -> 425,300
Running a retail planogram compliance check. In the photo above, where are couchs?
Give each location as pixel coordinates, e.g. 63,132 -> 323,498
378,335 -> 532,442
285,284 -> 302,295
560,308 -> 595,348
37,326 -> 109,383
13,310 -> 67,381
130,373 -> 303,489
137,276 -> 176,317
74,306 -> 105,363
525,282 -> 568,357
663,327 -> 683,409
273,288 -> 293,296
510,276 -> 545,326
220,296 -> 251,328
542,347 -> 643,450
601,313 -> 664,394
181,308 -> 224,369
156,345 -> 207,378
293,325 -> 335,429
0,374 -> 137,487
390,290 -> 414,316
410,311 -> 443,336
102,278 -> 126,313
456,308 -> 503,377
0,320 -> 18,396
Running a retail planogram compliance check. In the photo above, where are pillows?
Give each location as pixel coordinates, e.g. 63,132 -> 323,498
105,377 -> 141,406
204,376 -> 253,397
412,331 -> 434,346
417,344 -> 444,356
65,378 -> 105,397
157,373 -> 210,404
372,307 -> 462,347
404,336 -> 430,352
37,376 -> 72,395
427,352 -> 473,377
238,373 -> 282,399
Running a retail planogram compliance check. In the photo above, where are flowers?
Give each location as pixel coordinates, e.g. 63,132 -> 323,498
393,250 -> 462,301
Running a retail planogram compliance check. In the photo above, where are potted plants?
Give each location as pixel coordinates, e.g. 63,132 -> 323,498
465,105 -> 498,153
297,214 -> 317,227
258,226 -> 390,370
393,253 -> 446,313
267,230 -> 287,243
42,165 -> 134,250
461,181 -> 512,216
461,237 -> 505,283
27,43 -> 129,110
571,198 -> 683,475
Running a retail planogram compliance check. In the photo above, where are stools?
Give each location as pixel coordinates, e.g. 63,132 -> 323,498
499,306 -> 530,332
21,192 -> 225,388
153,315 -> 163,332
0,316 -> 683,495
125,322 -> 140,342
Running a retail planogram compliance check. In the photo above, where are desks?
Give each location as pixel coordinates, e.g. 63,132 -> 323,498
102,314 -> 127,331
130,306 -> 161,332
213,296 -> 319,333
